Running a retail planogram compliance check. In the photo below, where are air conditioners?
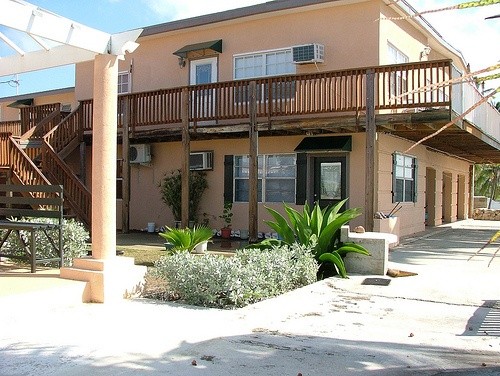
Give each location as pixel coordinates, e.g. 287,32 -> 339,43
291,43 -> 324,64
129,144 -> 151,165
188,152 -> 212,171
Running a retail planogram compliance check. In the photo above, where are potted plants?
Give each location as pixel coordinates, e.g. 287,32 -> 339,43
160,171 -> 201,230
220,193 -> 232,238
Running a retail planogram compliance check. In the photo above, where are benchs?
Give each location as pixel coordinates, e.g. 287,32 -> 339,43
0,183 -> 65,274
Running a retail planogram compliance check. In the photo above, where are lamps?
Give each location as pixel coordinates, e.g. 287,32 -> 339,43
178,56 -> 185,69
421,44 -> 431,57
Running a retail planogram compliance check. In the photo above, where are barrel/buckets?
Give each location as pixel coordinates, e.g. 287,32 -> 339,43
195,241 -> 208,254
147,222 -> 156,233
240,230 -> 249,239
